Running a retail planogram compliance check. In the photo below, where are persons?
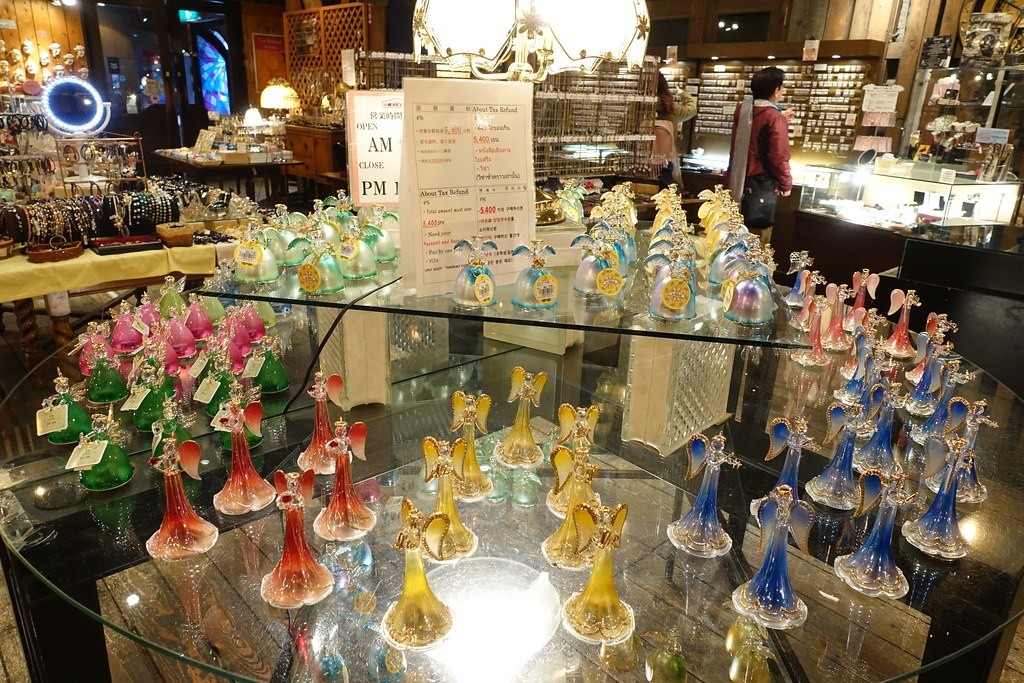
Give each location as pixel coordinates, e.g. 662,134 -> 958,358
145,370 -> 378,609
232,189 -> 399,297
42,259 -> 292,491
723,67 -> 796,248
449,178 -> 779,326
380,366 -> 635,651
615,71 -> 696,189
666,250 -> 999,629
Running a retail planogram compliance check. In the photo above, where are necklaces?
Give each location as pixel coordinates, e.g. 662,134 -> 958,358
0,191 -> 172,241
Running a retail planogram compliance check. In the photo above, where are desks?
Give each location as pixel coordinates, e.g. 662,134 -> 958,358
155,149 -> 305,209
0,237 -> 238,389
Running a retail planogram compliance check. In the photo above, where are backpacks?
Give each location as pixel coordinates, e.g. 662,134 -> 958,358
637,120 -> 676,163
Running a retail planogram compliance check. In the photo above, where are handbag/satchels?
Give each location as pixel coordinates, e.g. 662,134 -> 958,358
741,174 -> 779,228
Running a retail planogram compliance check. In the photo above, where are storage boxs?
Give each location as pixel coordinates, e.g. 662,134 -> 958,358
155,216 -> 262,247
212,149 -> 272,165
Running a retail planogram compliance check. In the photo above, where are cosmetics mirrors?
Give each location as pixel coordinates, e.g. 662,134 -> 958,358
858,149 -> 877,166
31,75 -> 111,184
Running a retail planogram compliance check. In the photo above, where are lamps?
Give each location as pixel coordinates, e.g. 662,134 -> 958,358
244,108 -> 265,153
411,0 -> 650,223
260,77 -> 300,125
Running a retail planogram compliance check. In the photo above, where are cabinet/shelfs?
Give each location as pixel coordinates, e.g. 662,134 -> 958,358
314,128 -> 346,186
0,221 -> 1024,683
284,126 -> 314,178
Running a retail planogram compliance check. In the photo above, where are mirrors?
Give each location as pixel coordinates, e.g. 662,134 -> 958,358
37,76 -> 111,187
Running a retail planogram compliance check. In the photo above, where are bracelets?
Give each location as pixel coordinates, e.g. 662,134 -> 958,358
0,114 -> 127,198
